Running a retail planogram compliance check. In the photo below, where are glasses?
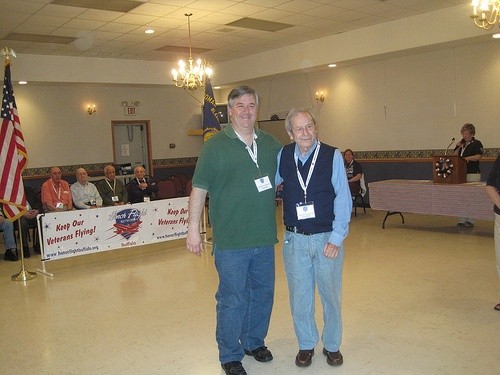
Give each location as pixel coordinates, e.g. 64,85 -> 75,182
291,124 -> 313,133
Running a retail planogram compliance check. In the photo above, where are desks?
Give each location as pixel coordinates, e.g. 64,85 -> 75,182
368,177 -> 494,230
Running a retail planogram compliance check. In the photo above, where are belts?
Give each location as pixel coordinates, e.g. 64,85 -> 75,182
286,226 -> 332,235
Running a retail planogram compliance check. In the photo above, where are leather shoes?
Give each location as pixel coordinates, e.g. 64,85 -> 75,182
221,361 -> 248,375
245,346 -> 273,362
322,348 -> 343,366
295,348 -> 314,367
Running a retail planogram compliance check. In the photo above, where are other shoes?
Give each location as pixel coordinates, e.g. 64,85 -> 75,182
23,244 -> 30,258
4,249 -> 18,261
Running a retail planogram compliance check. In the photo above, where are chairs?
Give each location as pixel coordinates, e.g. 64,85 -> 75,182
0,172 -> 210,254
349,172 -> 368,218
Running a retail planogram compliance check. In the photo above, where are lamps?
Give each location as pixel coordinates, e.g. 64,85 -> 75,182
171,12 -> 213,91
315,90 -> 324,105
87,103 -> 97,115
469,0 -> 500,32
120,100 -> 141,116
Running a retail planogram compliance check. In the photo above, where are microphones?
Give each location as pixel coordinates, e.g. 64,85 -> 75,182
444,138 -> 455,156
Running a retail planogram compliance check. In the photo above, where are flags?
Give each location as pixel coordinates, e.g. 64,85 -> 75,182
203,75 -> 221,145
0,56 -> 28,223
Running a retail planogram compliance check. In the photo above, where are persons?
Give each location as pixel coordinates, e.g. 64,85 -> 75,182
456,122 -> 483,231
127,166 -> 158,204
41,167 -> 76,212
275,107 -> 353,367
95,165 -> 128,206
186,85 -> 285,375
343,149 -> 362,208
0,202 -> 19,262
70,168 -> 103,209
485,156 -> 500,310
15,184 -> 44,258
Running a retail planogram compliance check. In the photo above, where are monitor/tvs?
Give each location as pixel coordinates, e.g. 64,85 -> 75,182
201,104 -> 229,126
